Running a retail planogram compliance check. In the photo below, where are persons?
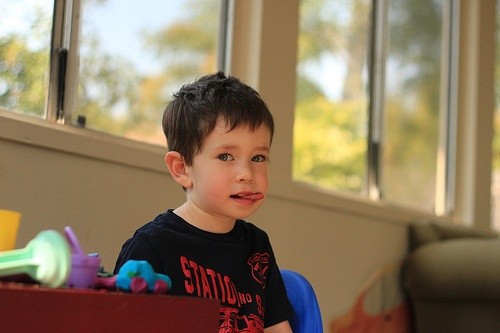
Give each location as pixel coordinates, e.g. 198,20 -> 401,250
114,71 -> 297,332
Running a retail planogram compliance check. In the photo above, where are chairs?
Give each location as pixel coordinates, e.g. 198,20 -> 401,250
280,269 -> 324,333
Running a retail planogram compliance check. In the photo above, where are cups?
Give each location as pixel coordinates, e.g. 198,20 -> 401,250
64,226 -> 101,289
0,208 -> 21,252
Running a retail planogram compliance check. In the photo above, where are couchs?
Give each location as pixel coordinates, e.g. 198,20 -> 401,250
398,222 -> 500,333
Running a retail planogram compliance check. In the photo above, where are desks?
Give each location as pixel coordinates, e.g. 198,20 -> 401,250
0,281 -> 221,333
